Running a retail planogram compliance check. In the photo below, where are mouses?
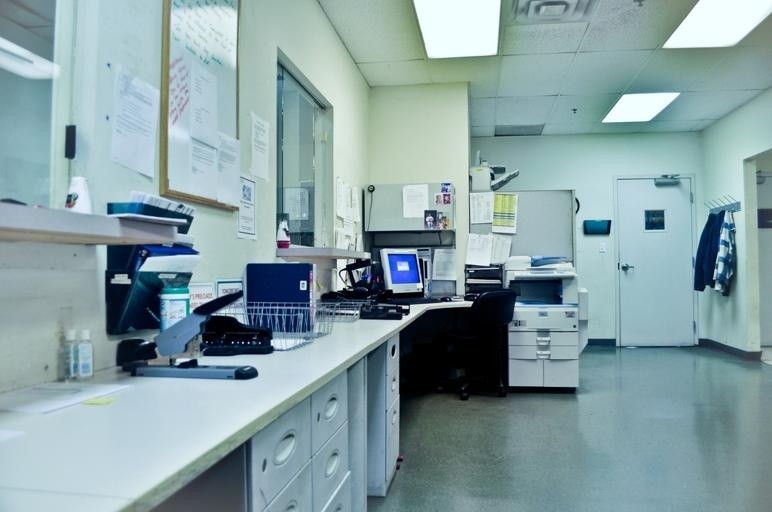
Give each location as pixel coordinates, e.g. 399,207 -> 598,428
440,296 -> 452,302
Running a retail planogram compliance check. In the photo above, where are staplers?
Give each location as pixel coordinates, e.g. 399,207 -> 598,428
116,290 -> 259,378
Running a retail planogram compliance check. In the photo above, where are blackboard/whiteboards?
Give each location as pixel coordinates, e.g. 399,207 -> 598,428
159,0 -> 241,212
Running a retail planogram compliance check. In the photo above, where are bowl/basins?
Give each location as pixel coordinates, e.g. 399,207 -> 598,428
277,240 -> 290,248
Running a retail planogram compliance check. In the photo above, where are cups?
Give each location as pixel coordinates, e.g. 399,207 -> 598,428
64,175 -> 92,214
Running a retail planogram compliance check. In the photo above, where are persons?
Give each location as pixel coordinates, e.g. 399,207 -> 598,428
425,183 -> 450,228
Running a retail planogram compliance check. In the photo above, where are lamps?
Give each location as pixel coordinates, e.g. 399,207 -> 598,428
338,259 -> 373,299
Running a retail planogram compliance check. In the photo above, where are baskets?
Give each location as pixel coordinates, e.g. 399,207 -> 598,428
213,301 -> 362,351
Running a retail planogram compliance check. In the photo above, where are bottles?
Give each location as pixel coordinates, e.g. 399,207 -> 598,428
159,288 -> 190,333
61,329 -> 95,384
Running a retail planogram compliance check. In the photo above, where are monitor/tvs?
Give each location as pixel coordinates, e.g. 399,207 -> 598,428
379,247 -> 423,294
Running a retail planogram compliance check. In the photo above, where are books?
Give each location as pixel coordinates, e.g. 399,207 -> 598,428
243,259 -> 320,333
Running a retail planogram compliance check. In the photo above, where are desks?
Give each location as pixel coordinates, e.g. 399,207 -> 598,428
0,293 -> 474,512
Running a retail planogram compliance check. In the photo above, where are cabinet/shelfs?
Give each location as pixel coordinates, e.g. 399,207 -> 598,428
506,308 -> 580,390
385,335 -> 400,497
248,369 -> 349,511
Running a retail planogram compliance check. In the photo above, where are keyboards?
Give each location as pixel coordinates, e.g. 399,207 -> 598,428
386,297 -> 439,304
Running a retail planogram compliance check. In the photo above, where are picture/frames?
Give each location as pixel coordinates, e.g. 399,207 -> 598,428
642,208 -> 668,233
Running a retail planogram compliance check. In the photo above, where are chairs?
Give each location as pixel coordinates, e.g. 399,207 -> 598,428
446,289 -> 518,401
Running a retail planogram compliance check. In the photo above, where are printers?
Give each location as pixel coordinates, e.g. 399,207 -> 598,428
502,254 -> 579,332
